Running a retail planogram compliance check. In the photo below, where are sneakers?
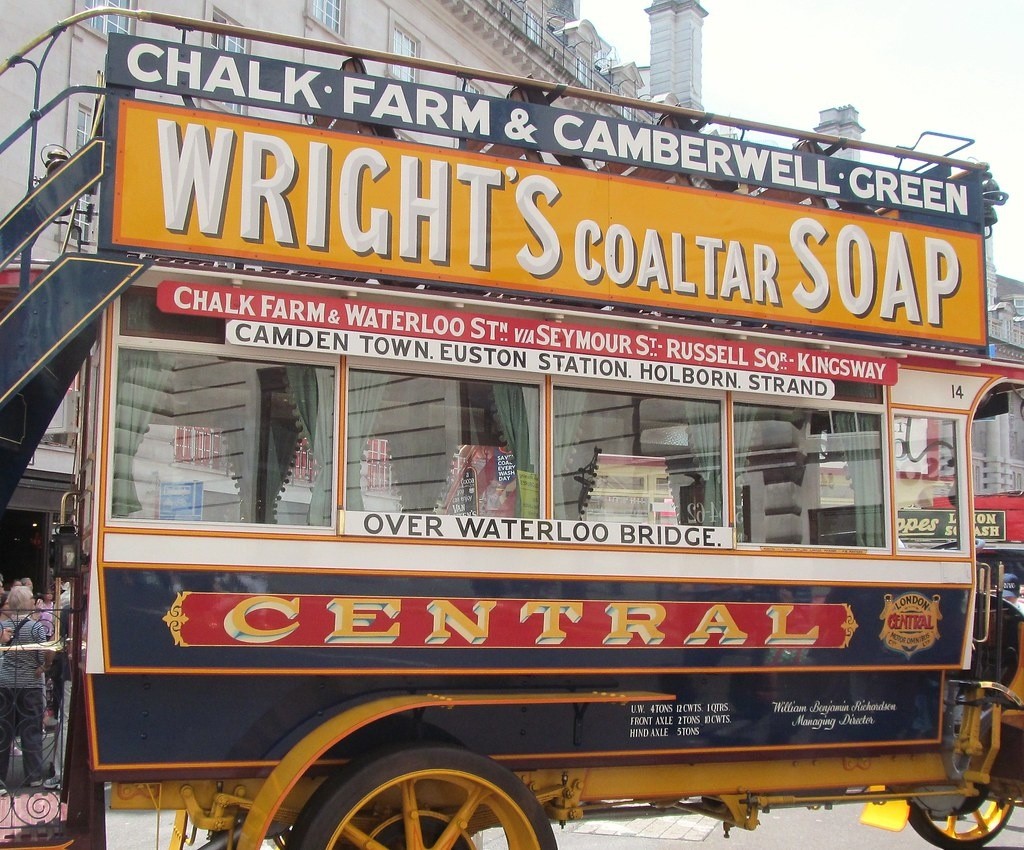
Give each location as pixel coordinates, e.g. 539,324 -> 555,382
43,776 -> 60,789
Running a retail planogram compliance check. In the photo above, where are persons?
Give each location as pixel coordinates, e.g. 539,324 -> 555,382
0,573 -> 73,796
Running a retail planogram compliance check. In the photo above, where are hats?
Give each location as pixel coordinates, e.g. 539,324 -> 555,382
61,582 -> 69,590
1001,572 -> 1020,598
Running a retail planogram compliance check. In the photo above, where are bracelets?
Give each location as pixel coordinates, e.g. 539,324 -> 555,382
45,662 -> 52,667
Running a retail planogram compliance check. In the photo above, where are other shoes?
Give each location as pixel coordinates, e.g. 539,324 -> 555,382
30,778 -> 42,786
0,781 -> 7,795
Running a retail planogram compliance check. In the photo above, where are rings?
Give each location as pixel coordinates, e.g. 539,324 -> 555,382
11,636 -> 14,638
